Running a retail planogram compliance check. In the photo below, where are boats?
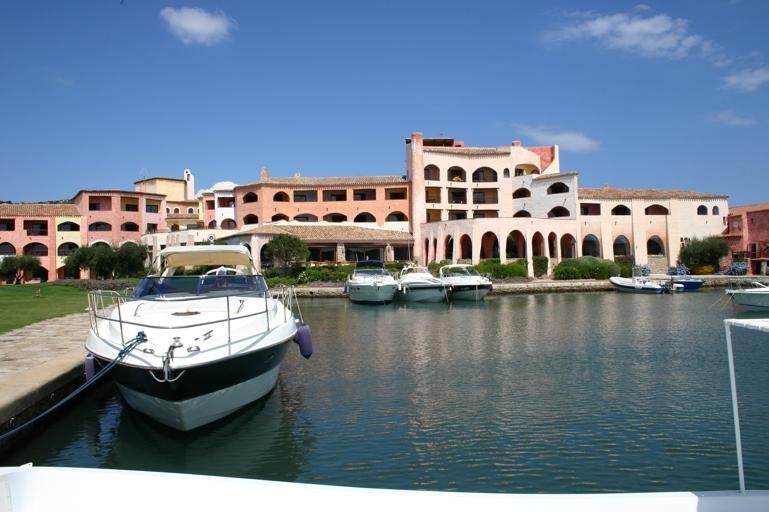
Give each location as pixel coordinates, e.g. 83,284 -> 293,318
438,264 -> 494,302
111,382 -> 282,479
82,245 -> 313,443
711,270 -> 769,312
394,238 -> 453,304
609,261 -> 672,294
664,282 -> 684,293
343,259 -> 399,306
663,279 -> 705,291
